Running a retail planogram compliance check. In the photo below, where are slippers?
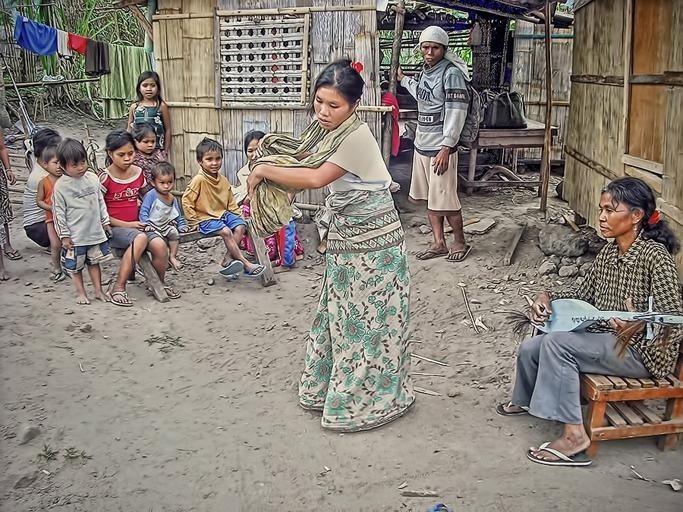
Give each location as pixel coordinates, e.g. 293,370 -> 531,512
52,271 -> 66,281
416,249 -> 449,260
448,243 -> 472,262
220,259 -> 244,275
149,286 -> 181,298
110,291 -> 134,306
526,441 -> 592,466
3,249 -> 22,260
243,264 -> 267,277
496,400 -> 530,416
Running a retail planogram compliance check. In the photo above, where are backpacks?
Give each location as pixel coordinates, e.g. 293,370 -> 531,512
416,63 -> 487,149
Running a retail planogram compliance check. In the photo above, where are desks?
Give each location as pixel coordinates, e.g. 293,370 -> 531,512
458,117 -> 560,197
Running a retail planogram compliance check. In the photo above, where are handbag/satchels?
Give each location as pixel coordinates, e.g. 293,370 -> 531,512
479,89 -> 527,129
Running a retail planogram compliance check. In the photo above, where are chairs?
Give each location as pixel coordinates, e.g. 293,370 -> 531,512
579,348 -> 683,457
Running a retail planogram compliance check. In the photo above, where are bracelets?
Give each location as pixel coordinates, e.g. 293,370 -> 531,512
5,168 -> 13,173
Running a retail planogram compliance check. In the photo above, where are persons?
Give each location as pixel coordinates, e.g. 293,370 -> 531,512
139,161 -> 184,272
0,76 -> 22,260
245,57 -> 416,433
236,131 -> 305,263
182,136 -> 266,278
50,137 -> 112,306
98,130 -> 181,307
273,219 -> 299,275
22,127 -> 61,254
36,142 -> 66,283
396,24 -> 471,260
132,121 -> 165,191
125,70 -> 172,159
500,176 -> 682,462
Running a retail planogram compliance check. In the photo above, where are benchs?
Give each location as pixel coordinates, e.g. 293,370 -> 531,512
110,220 -> 277,303
